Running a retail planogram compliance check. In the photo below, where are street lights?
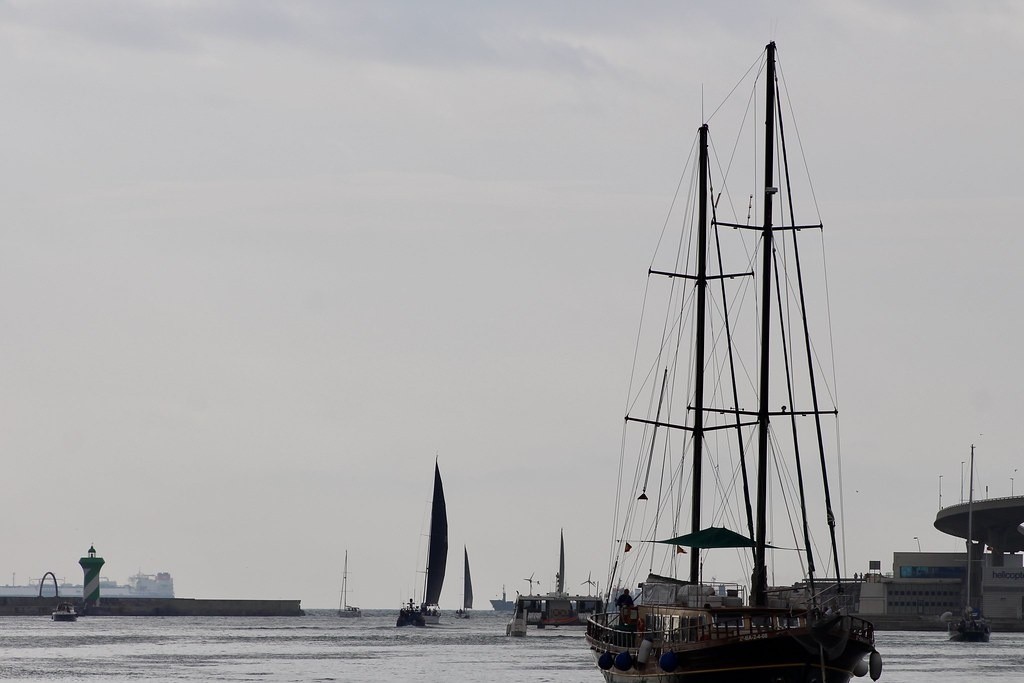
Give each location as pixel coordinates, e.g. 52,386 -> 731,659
938,474 -> 944,511
913,537 -> 921,552
1009,476 -> 1014,498
961,462 -> 966,504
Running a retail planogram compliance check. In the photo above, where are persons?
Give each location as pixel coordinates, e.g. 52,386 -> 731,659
617,589 -> 633,625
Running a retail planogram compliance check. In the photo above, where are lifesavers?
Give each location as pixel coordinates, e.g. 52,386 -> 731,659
553,609 -> 560,616
560,610 -> 567,617
635,618 -> 645,636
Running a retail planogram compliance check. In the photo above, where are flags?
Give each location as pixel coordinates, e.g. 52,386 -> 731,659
677,545 -> 687,555
625,543 -> 632,552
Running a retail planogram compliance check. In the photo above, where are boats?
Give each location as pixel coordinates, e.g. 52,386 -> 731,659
51,601 -> 78,622
488,584 -> 516,612
395,598 -> 426,627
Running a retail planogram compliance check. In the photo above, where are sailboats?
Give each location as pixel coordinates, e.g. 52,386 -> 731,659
452,544 -> 473,619
943,444 -> 993,642
580,36 -> 881,682
414,454 -> 450,625
337,549 -> 362,618
